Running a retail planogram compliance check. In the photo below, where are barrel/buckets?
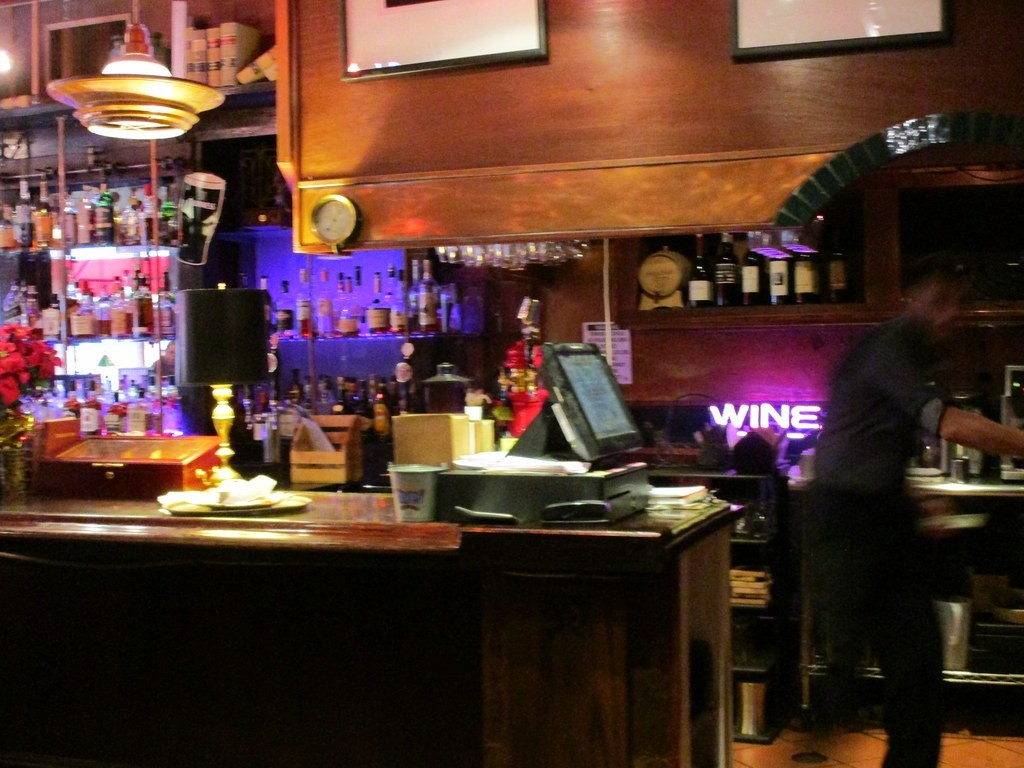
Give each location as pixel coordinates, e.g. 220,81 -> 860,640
638,252 -> 690,298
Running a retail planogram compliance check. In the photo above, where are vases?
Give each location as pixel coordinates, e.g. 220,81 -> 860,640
0,409 -> 28,506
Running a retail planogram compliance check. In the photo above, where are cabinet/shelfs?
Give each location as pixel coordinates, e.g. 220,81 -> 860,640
282,249 -> 541,416
0,166 -> 194,405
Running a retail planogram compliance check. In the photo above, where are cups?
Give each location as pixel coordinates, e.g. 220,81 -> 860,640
388,463 -> 446,523
950,457 -> 969,483
432,240 -> 587,271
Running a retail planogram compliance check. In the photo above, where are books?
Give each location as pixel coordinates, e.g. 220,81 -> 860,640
187,21 -> 276,88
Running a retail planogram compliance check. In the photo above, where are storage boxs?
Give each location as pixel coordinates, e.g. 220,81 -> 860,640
391,413 -> 495,465
199,226 -> 293,335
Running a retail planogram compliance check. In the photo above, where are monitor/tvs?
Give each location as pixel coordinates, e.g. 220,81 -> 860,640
541,341 -> 644,463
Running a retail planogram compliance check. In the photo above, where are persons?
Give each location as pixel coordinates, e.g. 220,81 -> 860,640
817,252 -> 1024,768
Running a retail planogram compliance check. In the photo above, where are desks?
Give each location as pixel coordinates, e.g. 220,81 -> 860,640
787,478 -> 1024,733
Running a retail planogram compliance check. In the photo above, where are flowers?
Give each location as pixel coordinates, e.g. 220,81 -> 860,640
0,326 -> 61,406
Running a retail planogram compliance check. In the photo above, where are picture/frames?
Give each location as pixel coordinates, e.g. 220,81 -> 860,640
339,0 -> 551,84
729,0 -> 954,63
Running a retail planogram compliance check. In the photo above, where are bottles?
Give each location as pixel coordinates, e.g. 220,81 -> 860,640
239,372 -> 416,442
0,179 -> 179,252
258,258 -> 439,337
3,268 -> 175,339
687,232 -> 850,308
18,373 -> 183,438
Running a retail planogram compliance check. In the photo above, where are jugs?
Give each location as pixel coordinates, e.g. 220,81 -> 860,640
934,596 -> 972,671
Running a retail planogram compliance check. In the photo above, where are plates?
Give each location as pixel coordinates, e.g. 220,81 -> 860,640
163,490 -> 313,515
907,468 -> 944,476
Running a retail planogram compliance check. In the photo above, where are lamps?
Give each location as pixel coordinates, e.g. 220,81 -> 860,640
46,0 -> 228,142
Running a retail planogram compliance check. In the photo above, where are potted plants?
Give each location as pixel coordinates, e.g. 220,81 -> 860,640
236,141 -> 286,224
